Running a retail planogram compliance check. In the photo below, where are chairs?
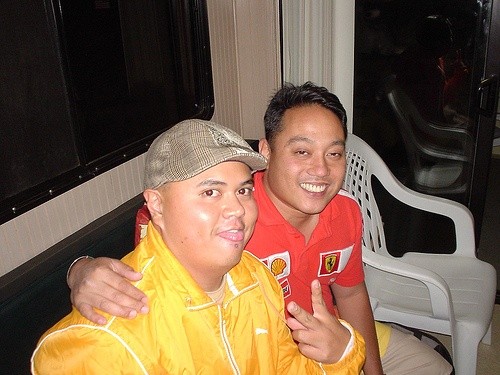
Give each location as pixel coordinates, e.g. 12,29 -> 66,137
336,133 -> 497,375
384,87 -> 471,199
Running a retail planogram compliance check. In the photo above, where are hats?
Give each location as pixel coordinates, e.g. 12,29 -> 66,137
143,118 -> 268,191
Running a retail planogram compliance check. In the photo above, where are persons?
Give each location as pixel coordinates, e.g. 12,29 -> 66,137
27,118 -> 367,375
62,81 -> 455,375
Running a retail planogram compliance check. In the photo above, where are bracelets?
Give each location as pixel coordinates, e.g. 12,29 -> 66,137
65,255 -> 95,288
